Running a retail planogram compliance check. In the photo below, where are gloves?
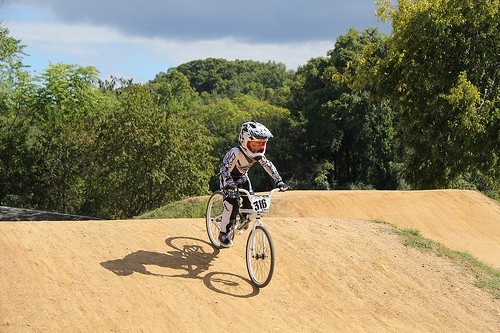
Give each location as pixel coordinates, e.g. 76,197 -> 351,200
227,187 -> 239,199
276,181 -> 288,192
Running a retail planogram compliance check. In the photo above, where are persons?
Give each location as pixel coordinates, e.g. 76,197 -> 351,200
218,122 -> 288,248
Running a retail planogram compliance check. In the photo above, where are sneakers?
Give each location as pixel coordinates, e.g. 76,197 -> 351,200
217,232 -> 234,248
240,219 -> 249,230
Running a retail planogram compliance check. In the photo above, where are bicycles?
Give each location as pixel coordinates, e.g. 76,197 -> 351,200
206,181 -> 290,288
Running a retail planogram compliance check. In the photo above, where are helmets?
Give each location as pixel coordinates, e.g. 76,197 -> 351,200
238,122 -> 274,162
